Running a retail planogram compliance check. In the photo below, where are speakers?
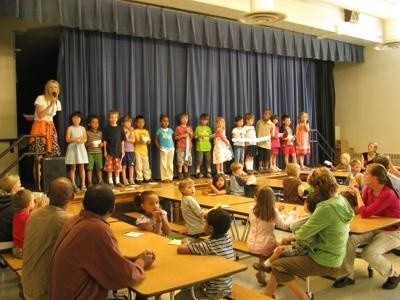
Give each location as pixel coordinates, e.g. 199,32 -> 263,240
41,155 -> 67,193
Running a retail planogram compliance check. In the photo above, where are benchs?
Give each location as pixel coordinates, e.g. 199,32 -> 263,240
123,212 -> 198,242
198,235 -> 351,300
16,269 -> 22,277
199,213 -> 400,278
1,253 -> 23,271
224,282 -> 274,300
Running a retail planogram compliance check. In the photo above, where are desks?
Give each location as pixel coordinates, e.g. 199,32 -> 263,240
153,185 -> 256,243
65,202 -> 119,223
302,170 -> 350,179
223,200 -> 400,242
256,177 -> 346,201
109,221 -> 248,300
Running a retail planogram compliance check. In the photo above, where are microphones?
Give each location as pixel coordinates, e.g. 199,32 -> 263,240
52,91 -> 58,106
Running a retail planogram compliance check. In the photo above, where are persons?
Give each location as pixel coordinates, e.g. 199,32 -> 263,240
48,183 -> 156,300
64,108 -> 311,193
134,178 -> 233,300
28,79 -> 62,191
248,143 -> 399,300
12,189 -> 47,259
0,174 -> 22,242
21,177 -> 75,300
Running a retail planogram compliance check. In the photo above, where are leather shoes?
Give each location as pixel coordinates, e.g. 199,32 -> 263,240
333,276 -> 355,288
383,273 -> 400,289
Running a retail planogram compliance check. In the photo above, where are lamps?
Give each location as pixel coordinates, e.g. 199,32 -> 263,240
240,0 -> 287,25
374,16 -> 399,51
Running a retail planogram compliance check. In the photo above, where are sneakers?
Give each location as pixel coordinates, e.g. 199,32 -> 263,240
137,180 -> 142,185
73,186 -> 79,193
124,180 -> 129,186
130,179 -> 135,185
115,183 -> 124,187
255,272 -> 266,284
253,263 -> 271,273
